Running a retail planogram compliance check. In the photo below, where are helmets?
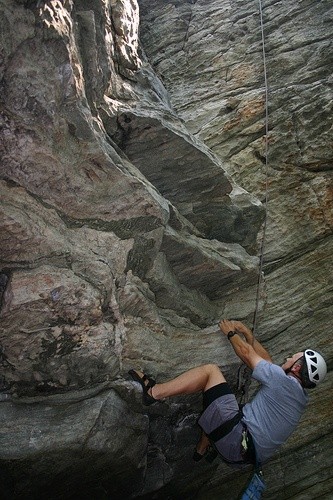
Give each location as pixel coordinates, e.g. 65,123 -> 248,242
304,349 -> 326,389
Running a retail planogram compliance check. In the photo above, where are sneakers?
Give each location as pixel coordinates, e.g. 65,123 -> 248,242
129,369 -> 158,409
192,441 -> 211,461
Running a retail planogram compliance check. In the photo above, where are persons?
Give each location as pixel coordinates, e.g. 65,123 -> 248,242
128,320 -> 328,463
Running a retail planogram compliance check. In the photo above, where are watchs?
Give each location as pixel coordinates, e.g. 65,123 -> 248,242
227,330 -> 237,340
